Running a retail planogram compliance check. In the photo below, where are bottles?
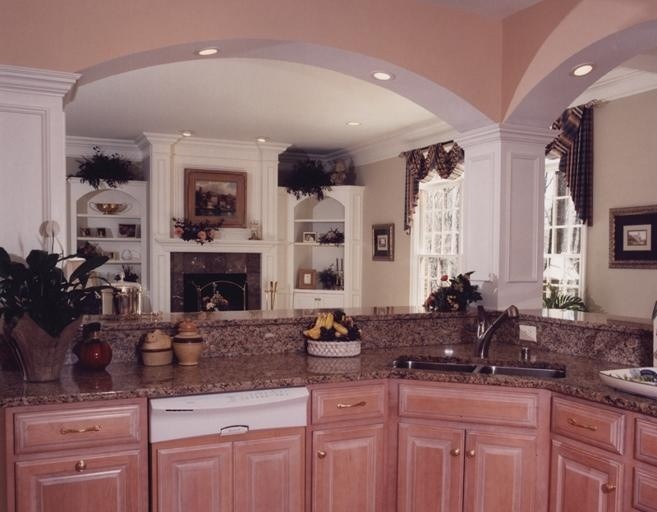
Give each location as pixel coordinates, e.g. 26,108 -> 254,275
82,322 -> 112,371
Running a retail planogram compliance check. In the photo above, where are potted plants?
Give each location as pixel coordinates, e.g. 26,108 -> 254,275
0,243 -> 121,382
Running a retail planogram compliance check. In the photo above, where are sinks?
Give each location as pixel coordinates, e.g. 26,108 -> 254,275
385,354 -> 476,372
478,360 -> 567,378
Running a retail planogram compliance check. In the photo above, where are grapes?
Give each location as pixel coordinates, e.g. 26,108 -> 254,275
319,326 -> 335,341
343,325 -> 360,341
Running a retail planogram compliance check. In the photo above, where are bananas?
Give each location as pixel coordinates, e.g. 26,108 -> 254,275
302,313 -> 349,339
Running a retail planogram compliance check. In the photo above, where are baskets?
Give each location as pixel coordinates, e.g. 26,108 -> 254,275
307,339 -> 361,358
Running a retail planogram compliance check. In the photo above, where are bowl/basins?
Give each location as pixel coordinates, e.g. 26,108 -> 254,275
96,203 -> 121,214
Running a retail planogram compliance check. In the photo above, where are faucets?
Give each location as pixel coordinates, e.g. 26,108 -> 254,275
473,303 -> 520,364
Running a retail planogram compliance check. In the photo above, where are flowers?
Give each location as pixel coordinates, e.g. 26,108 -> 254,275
172,218 -> 225,247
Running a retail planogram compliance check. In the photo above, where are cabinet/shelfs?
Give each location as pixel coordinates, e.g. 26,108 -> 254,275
150,387 -> 310,512
634,413 -> 656,512
3,396 -> 149,511
310,382 -> 397,512
397,379 -> 551,512
553,396 -> 625,512
67,175 -> 148,312
285,187 -> 365,313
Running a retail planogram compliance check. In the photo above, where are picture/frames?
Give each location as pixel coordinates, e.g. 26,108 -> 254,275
608,204 -> 657,270
303,232 -> 317,243
371,223 -> 395,262
183,168 -> 247,229
298,269 -> 316,289
